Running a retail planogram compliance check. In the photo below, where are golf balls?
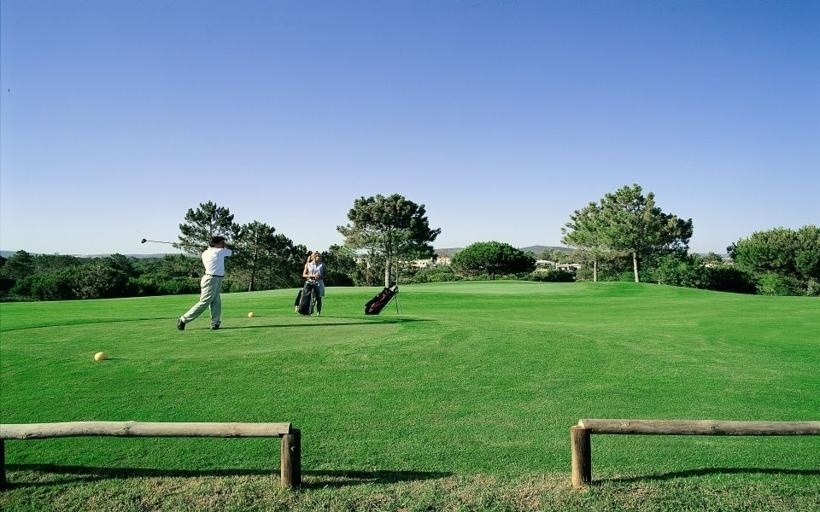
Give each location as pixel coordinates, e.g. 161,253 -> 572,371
94,352 -> 106,361
248,312 -> 255,318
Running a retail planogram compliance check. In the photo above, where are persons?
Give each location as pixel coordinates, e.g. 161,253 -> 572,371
177,236 -> 233,330
303,252 -> 327,316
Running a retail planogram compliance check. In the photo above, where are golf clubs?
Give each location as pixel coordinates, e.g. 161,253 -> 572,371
141,239 -> 209,248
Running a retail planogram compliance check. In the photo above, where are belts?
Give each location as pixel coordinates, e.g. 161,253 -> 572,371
204,273 -> 225,278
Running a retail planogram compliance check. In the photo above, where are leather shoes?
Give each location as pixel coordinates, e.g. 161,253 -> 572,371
177,317 -> 186,330
211,324 -> 220,329
